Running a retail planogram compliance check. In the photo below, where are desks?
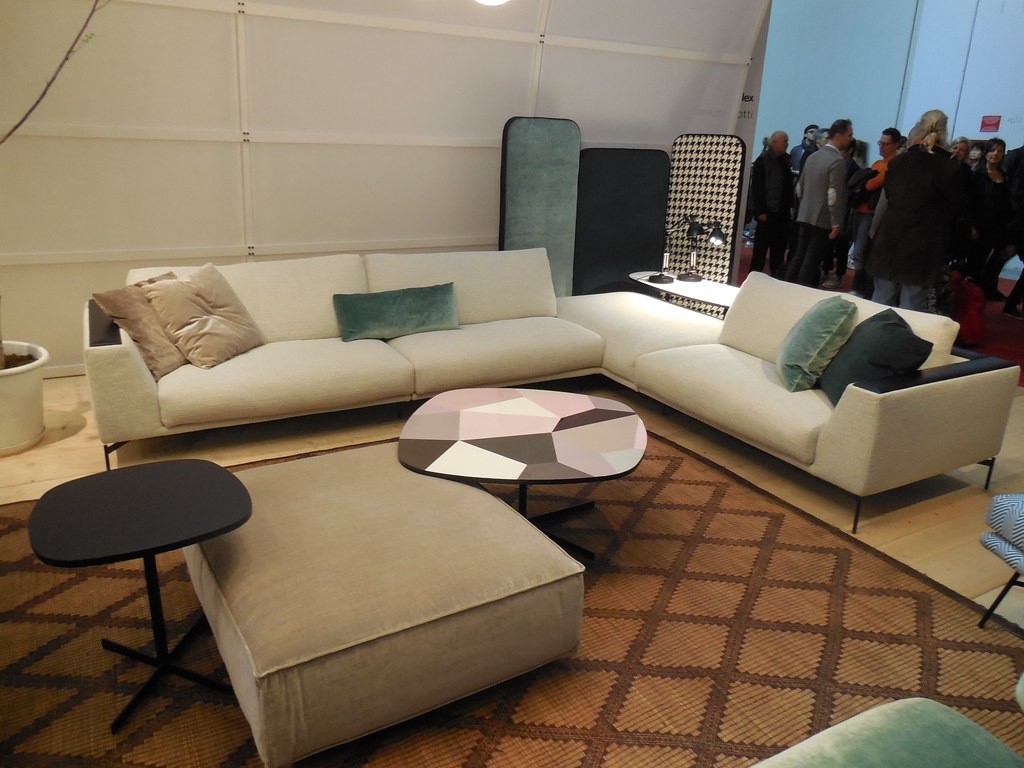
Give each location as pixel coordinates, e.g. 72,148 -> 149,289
397,388 -> 650,570
29,457 -> 253,736
628,269 -> 741,318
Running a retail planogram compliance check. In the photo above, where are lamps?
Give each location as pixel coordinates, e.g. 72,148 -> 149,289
647,214 -> 728,286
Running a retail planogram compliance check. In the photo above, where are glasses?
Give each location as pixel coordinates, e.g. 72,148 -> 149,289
878,141 -> 894,147
806,131 -> 815,134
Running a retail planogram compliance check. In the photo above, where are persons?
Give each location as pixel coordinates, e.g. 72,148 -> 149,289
743,110 -> 1024,321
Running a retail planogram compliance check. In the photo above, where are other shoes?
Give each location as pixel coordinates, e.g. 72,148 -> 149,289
1002,303 -> 1024,320
848,290 -> 869,299
990,290 -> 1006,301
822,274 -> 841,289
846,258 -> 856,270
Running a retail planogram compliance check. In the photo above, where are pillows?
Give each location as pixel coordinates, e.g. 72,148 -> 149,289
817,307 -> 934,411
332,281 -> 464,342
142,261 -> 272,370
775,295 -> 859,393
93,271 -> 193,384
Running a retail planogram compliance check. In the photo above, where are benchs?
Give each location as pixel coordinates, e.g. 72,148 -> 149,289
555,247 -> 1021,535
80,245 -> 607,470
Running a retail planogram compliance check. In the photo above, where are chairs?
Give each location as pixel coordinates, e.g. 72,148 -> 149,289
976,492 -> 1024,631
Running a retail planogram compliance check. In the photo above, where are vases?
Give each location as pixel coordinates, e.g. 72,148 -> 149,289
0,341 -> 50,459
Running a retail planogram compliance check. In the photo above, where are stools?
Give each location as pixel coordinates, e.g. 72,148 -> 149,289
181,439 -> 586,768
